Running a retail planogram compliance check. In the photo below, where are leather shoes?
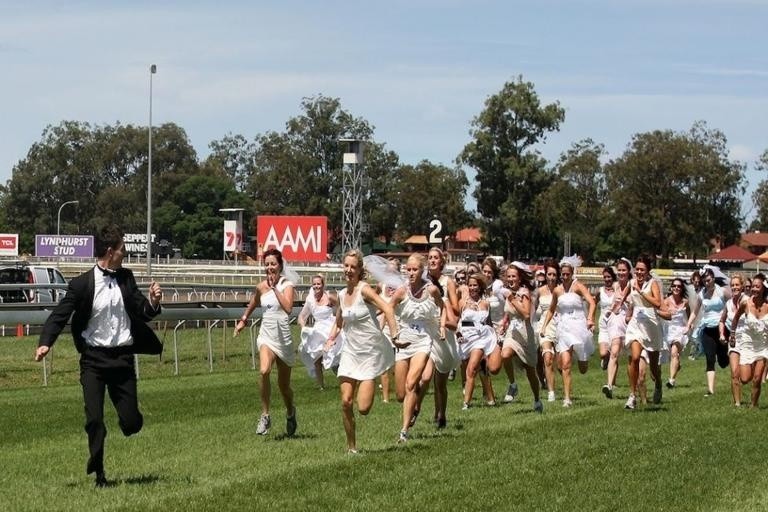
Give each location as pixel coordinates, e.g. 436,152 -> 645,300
96,473 -> 108,488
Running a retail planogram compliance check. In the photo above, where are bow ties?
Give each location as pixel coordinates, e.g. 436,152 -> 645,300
103,269 -> 116,279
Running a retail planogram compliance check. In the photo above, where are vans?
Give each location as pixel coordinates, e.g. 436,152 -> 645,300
0,259 -> 69,305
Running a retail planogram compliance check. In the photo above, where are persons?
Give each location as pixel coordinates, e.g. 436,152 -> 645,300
379,253 -> 447,443
594,258 -> 672,408
373,257 -> 409,403
324,248 -> 411,452
35,224 -> 163,484
300,274 -> 342,390
664,265 -> 768,409
410,248 -> 596,430
298,247 -> 768,453
234,249 -> 298,435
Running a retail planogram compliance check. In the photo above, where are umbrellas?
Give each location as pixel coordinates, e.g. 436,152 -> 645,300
707,244 -> 758,263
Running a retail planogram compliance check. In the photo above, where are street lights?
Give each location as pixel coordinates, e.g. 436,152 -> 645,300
145,64 -> 157,274
56,199 -> 81,266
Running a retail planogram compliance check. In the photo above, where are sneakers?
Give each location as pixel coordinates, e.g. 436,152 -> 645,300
447,368 -> 456,381
548,391 -> 556,401
256,414 -> 271,435
666,382 -> 673,388
504,383 -> 518,402
286,407 -> 296,436
534,400 -> 543,414
601,359 -> 608,370
704,391 -> 712,397
624,397 -> 636,409
602,385 -> 612,398
400,431 -> 414,442
562,400 -> 573,408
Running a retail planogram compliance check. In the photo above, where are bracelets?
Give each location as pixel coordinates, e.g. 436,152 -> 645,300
456,332 -> 462,339
240,318 -> 248,325
508,295 -> 516,302
391,336 -> 397,343
640,291 -> 643,295
